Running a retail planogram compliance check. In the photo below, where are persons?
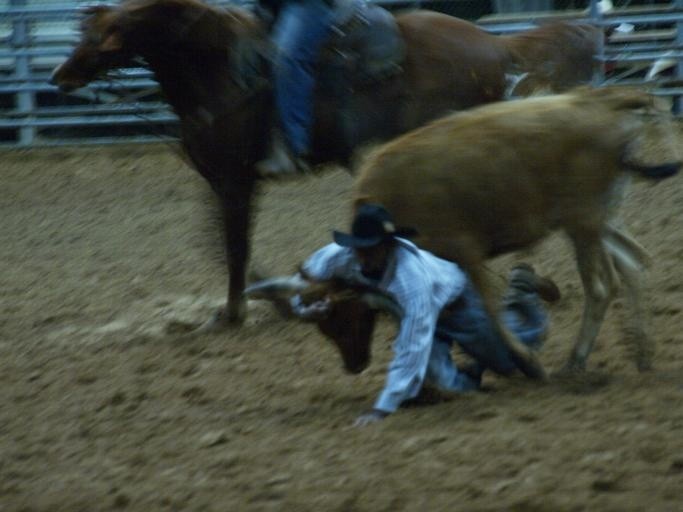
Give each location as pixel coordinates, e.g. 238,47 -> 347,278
254,3 -> 358,179
290,206 -> 559,430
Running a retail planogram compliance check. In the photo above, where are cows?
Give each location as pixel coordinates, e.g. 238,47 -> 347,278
241,85 -> 683,381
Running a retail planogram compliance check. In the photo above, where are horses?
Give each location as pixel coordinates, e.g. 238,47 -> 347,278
47,0 -> 606,339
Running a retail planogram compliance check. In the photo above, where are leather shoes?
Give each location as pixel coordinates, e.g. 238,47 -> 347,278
458,363 -> 480,381
510,262 -> 560,303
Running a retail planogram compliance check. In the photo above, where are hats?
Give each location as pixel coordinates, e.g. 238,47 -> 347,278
333,204 -> 417,249
